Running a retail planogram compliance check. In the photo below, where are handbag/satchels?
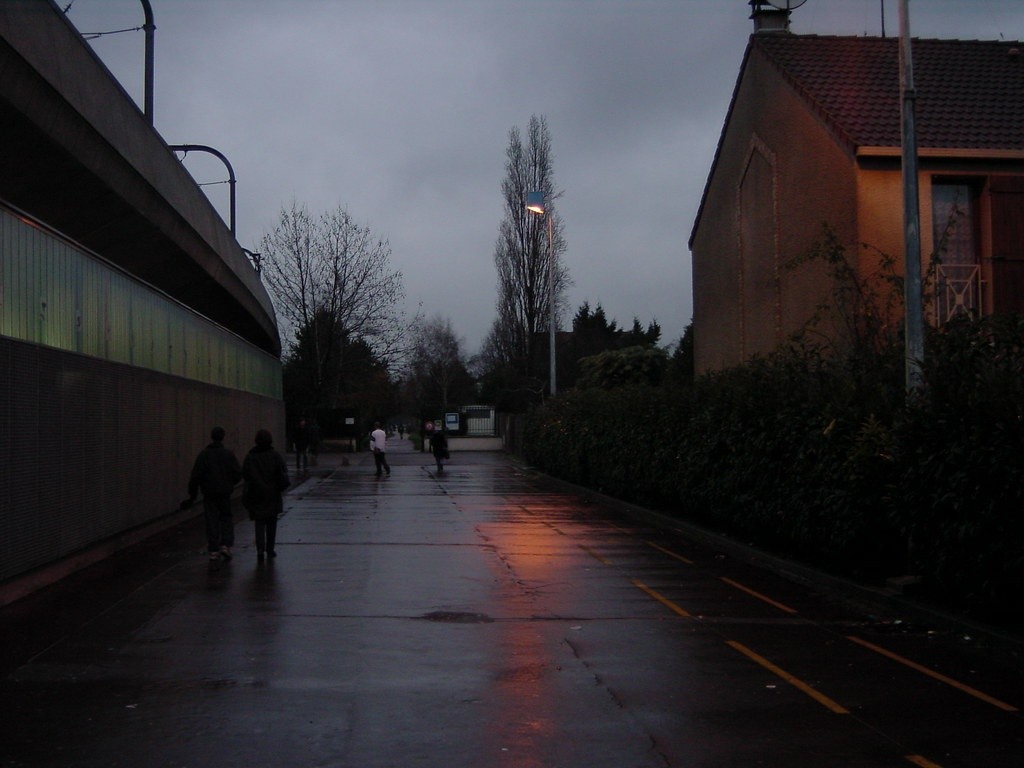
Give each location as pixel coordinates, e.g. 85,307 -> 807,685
373,447 -> 379,455
443,449 -> 449,459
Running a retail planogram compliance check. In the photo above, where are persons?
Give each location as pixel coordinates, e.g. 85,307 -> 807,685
369,421 -> 390,478
428,431 -> 447,472
292,419 -> 312,470
188,427 -> 240,561
242,429 -> 291,562
397,424 -> 404,440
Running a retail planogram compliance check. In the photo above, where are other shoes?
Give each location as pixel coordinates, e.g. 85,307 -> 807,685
221,546 -> 231,557
209,552 -> 220,561
257,550 -> 276,556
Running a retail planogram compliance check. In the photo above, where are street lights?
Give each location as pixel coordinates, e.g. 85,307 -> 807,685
527,190 -> 558,396
